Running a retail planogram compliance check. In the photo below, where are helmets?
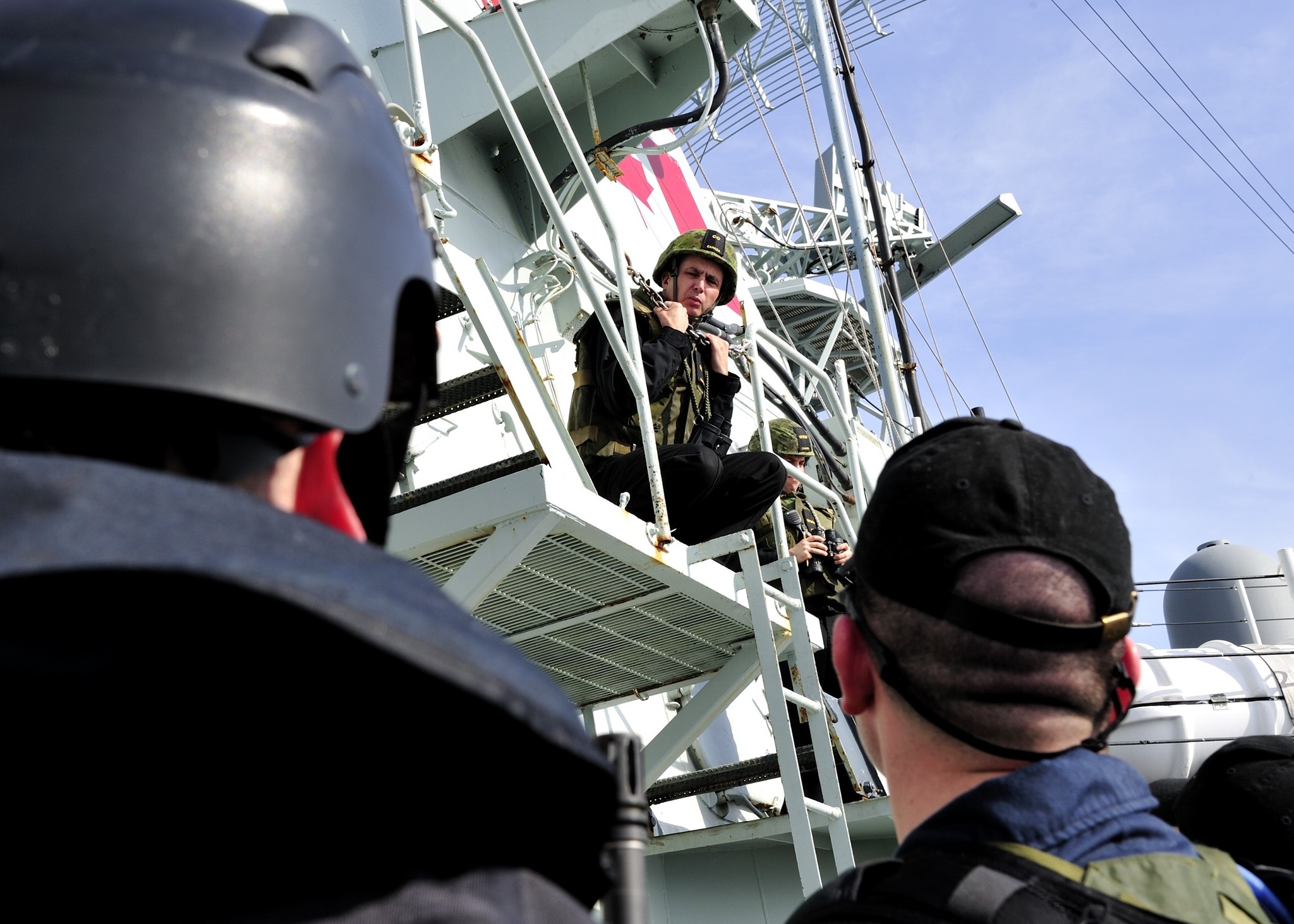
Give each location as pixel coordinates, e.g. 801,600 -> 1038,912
747,418 -> 815,458
652,229 -> 738,306
0,0 -> 444,436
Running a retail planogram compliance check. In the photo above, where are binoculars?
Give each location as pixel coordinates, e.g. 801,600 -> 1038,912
806,528 -> 850,575
690,412 -> 732,457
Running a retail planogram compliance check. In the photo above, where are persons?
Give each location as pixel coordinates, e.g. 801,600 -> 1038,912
790,414 -> 1294,923
1149,735 -> 1294,923
736,416 -> 854,613
568,229 -> 791,568
0,1 -> 628,924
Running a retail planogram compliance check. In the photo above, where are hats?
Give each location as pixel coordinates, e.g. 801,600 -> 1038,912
854,417 -> 1134,650
1147,735 -> 1294,924
